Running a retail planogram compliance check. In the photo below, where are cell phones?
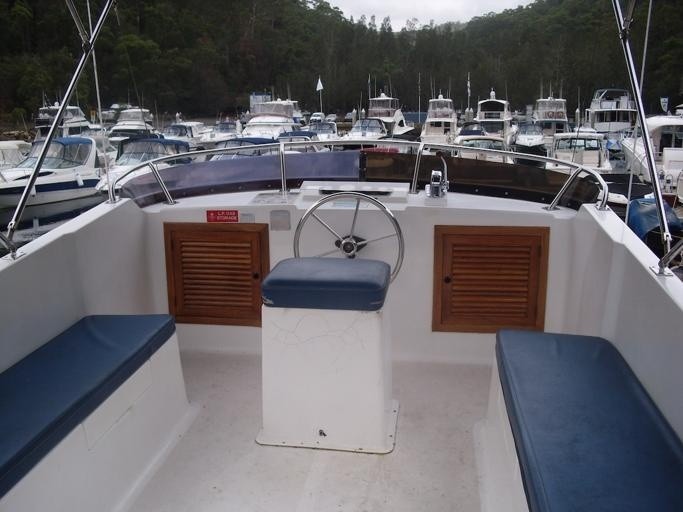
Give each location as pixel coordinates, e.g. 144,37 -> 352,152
430,170 -> 441,197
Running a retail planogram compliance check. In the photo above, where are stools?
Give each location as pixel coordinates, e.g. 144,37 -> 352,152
255,259 -> 399,454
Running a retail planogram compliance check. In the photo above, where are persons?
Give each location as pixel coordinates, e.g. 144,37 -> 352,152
175,109 -> 183,122
380,88 -> 388,97
488,86 -> 496,99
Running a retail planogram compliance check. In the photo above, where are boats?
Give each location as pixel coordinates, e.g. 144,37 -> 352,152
620,97 -> 683,205
87,102 -> 140,131
158,112 -> 205,139
244,69 -> 417,157
0,135 -> 116,229
450,72 -> 491,150
475,88 -> 519,149
531,77 -> 569,136
27,90 -> 106,145
191,138 -> 280,164
546,86 -> 612,177
108,105 -> 155,135
628,197 -> 683,263
583,89 -> 640,163
192,114 -> 244,149
93,131 -> 208,197
514,119 -> 547,164
419,76 -> 463,151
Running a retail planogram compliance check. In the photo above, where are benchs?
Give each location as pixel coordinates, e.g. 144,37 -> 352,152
471,330 -> 682,512
0,315 -> 203,511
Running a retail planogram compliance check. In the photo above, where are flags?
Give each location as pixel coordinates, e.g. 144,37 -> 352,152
466,73 -> 472,97
315,75 -> 324,92
